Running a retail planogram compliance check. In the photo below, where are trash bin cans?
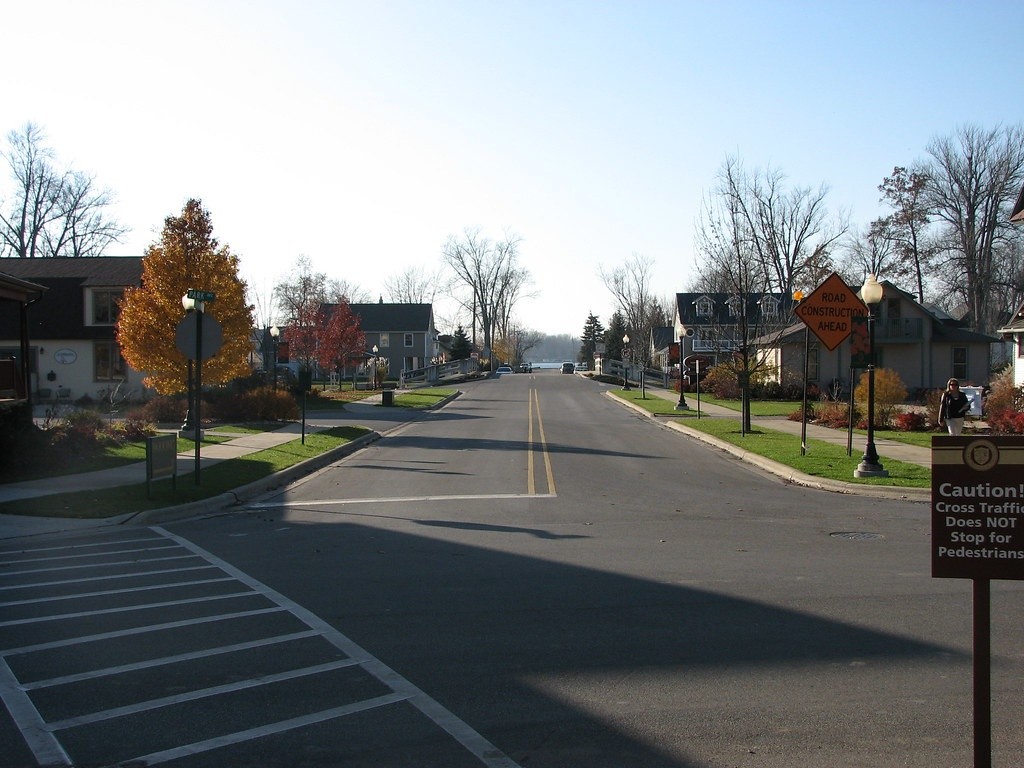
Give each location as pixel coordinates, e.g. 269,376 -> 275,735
381,390 -> 395,405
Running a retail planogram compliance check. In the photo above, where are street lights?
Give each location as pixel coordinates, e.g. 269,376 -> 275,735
177,287 -> 196,428
676,323 -> 688,405
270,324 -> 281,398
861,273 -> 884,461
623,333 -> 631,389
372,345 -> 379,391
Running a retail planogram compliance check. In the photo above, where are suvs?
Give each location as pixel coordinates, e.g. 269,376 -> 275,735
520,363 -> 532,373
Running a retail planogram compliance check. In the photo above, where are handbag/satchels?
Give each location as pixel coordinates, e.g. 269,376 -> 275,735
942,391 -> 951,419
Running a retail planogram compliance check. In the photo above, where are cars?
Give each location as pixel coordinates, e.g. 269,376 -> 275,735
495,366 -> 515,375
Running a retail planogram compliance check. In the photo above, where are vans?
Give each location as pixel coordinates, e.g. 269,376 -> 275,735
560,363 -> 575,374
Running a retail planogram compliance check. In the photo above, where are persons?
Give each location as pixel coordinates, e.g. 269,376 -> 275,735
938,378 -> 970,435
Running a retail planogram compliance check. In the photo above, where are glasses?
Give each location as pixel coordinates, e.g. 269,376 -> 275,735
950,384 -> 958,386
